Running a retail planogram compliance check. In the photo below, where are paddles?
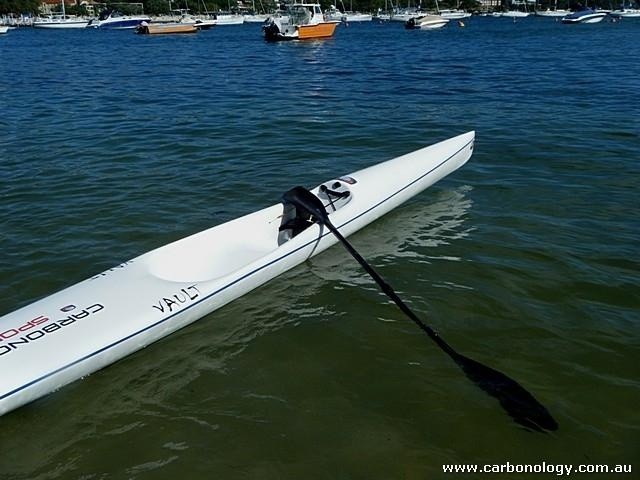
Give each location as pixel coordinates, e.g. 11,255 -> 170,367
283,186 -> 558,430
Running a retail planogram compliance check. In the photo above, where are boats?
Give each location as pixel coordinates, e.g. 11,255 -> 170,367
0,129 -> 475,418
